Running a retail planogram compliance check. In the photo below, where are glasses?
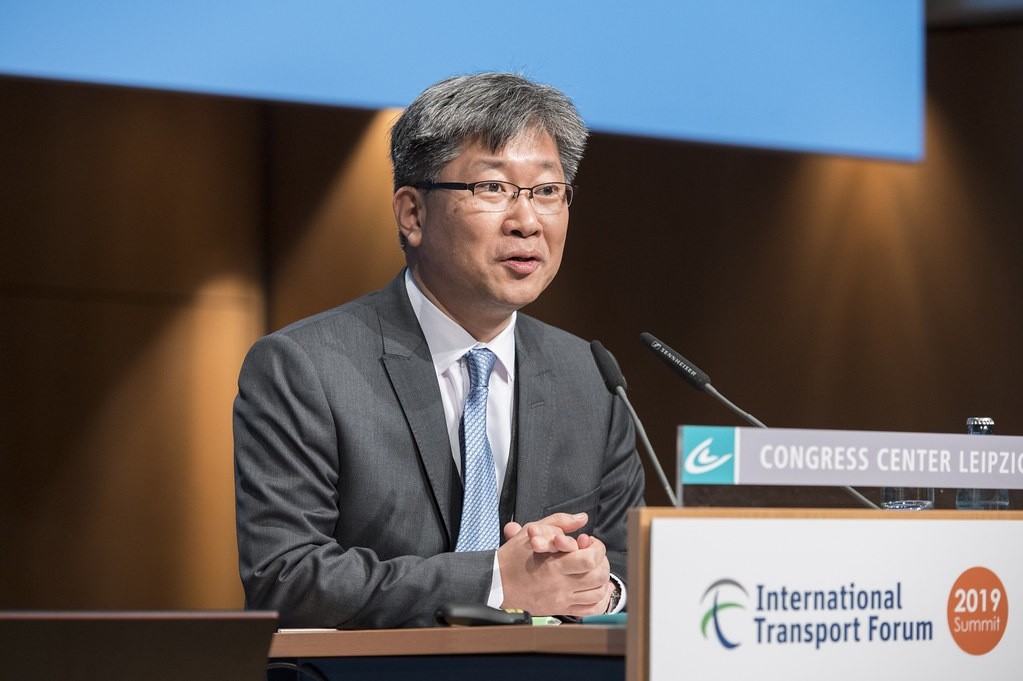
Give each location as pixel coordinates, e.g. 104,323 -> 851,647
413,181 -> 579,214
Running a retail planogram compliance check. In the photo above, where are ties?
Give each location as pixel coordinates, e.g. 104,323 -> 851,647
454,348 -> 500,552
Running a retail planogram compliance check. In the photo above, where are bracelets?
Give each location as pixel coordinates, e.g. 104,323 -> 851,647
607,590 -> 620,613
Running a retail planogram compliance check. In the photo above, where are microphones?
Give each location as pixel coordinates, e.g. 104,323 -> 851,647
639,334 -> 882,512
590,339 -> 677,510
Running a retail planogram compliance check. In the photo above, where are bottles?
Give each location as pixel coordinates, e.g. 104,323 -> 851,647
955,417 -> 1011,511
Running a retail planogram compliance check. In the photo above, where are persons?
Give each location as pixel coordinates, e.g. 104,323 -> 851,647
233,73 -> 650,630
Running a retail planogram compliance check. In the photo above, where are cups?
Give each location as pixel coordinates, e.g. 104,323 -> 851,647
878,488 -> 936,510
